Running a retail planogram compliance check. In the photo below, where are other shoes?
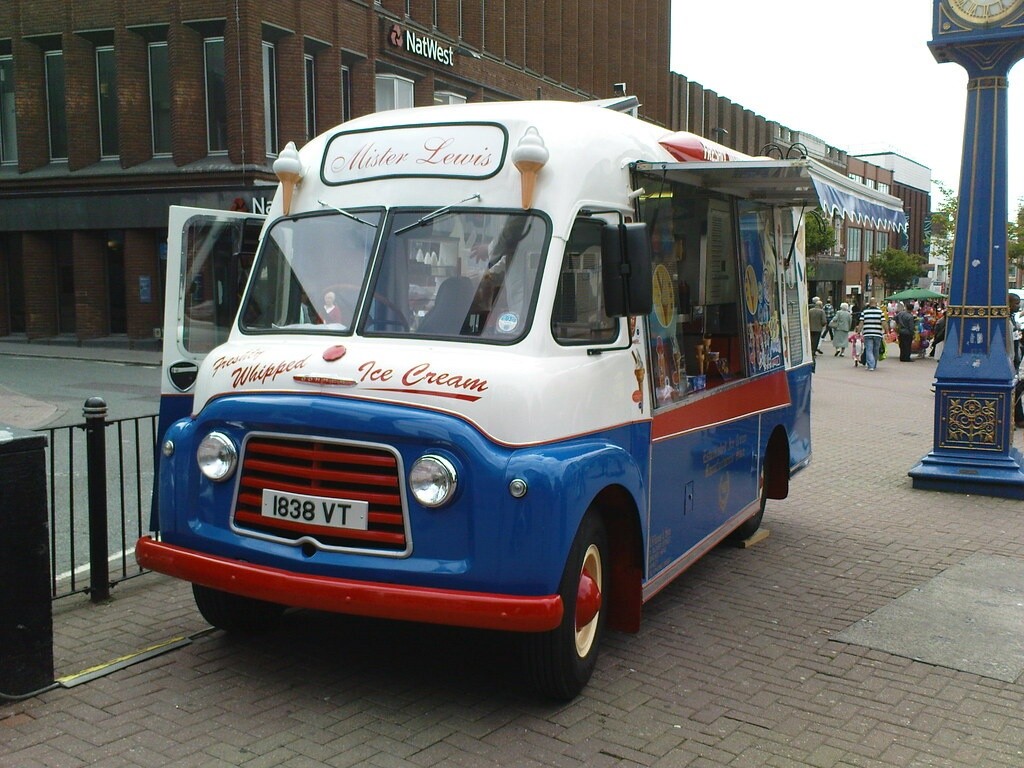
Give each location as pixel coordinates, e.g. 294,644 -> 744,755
855,360 -> 858,367
835,349 -> 840,356
1015,421 -> 1024,428
839,353 -> 844,357
864,368 -> 874,370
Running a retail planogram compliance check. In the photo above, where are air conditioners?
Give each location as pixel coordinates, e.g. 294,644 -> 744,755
522,249 -> 616,331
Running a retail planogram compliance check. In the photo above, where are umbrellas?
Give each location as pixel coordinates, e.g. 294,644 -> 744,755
884,288 -> 947,301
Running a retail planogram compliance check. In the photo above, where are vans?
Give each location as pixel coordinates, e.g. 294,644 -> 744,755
133,93 -> 908,707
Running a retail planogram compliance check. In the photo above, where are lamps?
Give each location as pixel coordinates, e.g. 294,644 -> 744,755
711,127 -> 729,135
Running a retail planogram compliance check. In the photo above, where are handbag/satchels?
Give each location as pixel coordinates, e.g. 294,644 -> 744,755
828,318 -> 838,329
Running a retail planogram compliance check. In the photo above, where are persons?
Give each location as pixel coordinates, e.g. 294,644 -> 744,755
315,292 -> 342,325
1008,292 -> 1024,428
808,295 -> 949,371
469,212 -> 533,320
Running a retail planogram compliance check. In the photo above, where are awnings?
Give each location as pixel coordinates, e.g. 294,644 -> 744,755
629,154 -> 910,235
